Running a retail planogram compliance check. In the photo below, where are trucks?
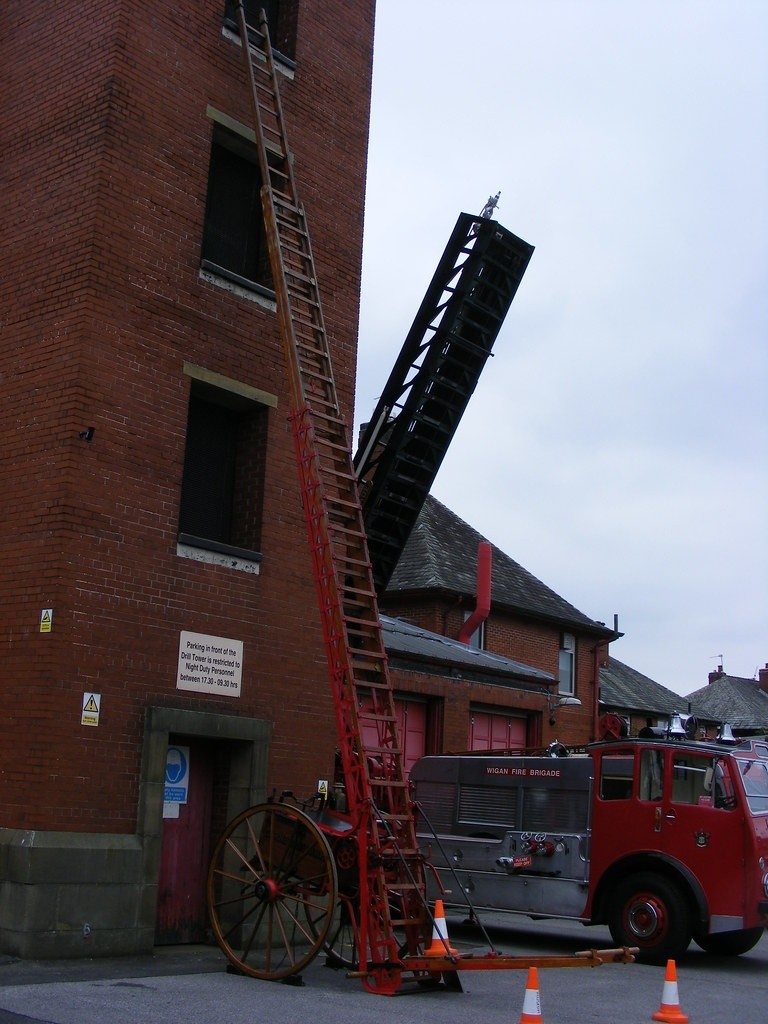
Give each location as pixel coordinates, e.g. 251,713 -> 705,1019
404,708 -> 767,965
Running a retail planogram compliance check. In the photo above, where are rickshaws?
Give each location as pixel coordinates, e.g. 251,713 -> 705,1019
208,784 -> 639,983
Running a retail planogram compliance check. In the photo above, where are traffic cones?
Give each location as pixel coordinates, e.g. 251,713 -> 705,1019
651,960 -> 688,1024
516,967 -> 544,1024
424,899 -> 459,957
